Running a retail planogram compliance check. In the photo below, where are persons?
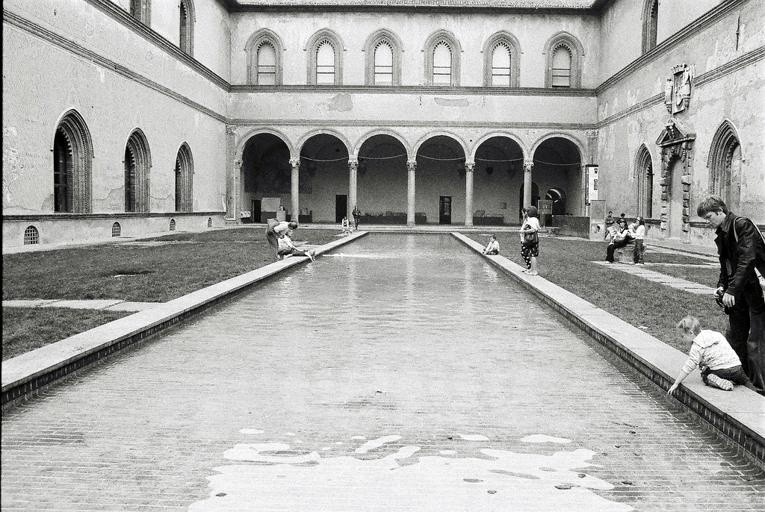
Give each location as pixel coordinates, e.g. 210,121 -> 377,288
666,315 -> 757,398
482,234 -> 500,255
697,196 -> 765,397
352,205 -> 361,230
519,206 -> 541,276
519,208 -> 531,272
265,204 -> 316,262
603,210 -> 645,264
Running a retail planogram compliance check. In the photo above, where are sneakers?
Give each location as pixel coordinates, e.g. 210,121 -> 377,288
707,373 -> 733,390
